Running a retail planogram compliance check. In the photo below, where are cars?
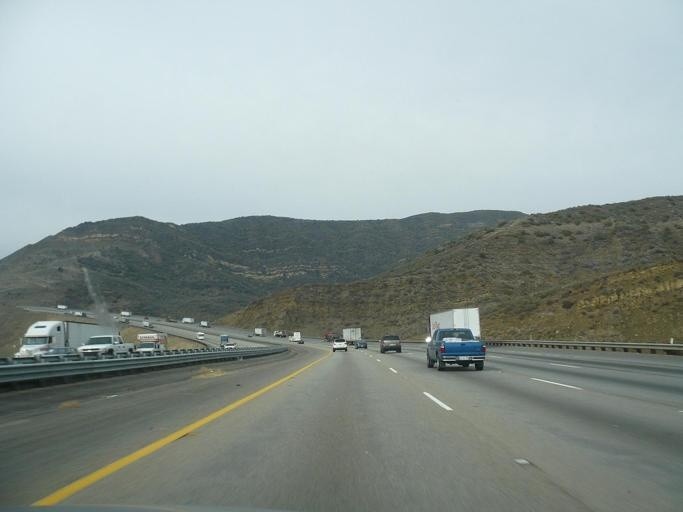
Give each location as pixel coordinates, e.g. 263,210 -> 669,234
247,326 -> 401,354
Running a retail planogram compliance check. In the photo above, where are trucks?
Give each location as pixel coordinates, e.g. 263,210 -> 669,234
425,307 -> 483,346
13,303 -> 237,366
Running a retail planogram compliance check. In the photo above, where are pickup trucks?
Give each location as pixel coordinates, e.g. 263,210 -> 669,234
423,327 -> 486,373
